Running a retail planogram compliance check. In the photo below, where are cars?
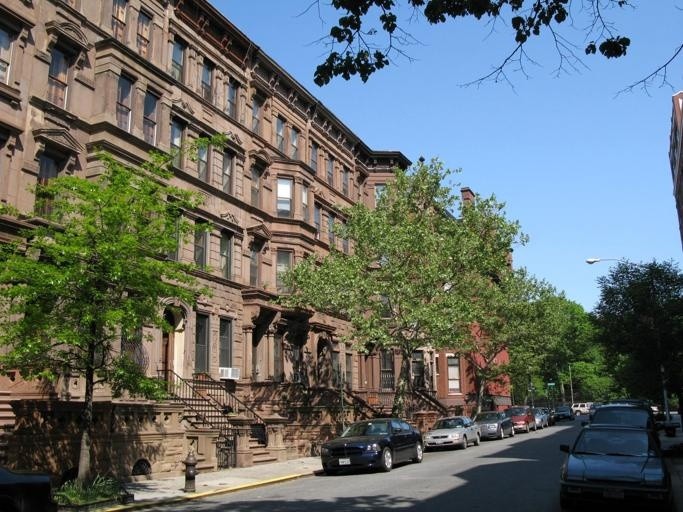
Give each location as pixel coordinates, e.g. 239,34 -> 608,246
560,400 -> 673,504
320,417 -> 424,475
557,402 -> 594,419
424,406 -> 555,449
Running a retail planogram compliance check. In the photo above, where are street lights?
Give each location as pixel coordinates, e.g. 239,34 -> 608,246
586,257 -> 671,422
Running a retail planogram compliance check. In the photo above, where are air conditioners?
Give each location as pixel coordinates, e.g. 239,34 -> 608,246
217,366 -> 240,379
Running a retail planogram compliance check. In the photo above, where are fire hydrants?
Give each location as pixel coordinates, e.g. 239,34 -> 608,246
183,451 -> 196,494
